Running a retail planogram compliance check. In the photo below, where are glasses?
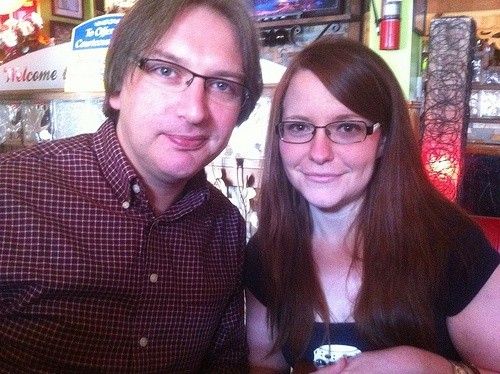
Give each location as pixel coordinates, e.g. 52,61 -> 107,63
273,120 -> 380,145
126,52 -> 252,109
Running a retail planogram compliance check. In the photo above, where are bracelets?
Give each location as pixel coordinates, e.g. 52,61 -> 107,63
463,361 -> 481,374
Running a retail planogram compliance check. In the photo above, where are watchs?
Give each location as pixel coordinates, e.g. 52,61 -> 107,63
450,359 -> 469,374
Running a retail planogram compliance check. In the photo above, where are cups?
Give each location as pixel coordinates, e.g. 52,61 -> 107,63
379,0 -> 403,50
314,345 -> 362,369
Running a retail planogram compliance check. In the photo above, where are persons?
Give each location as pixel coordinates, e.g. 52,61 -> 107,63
243,38 -> 500,374
1,1 -> 264,374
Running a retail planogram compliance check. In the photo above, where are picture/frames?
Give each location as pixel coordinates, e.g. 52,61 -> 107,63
413,0 -> 427,36
51,0 -> 84,20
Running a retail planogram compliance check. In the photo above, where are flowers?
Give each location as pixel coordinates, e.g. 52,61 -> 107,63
0,0 -> 50,64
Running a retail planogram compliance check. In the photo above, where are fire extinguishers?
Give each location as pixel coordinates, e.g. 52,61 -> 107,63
371,0 -> 401,50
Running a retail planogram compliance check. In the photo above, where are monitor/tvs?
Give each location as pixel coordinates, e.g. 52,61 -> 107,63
251,0 -> 345,21
